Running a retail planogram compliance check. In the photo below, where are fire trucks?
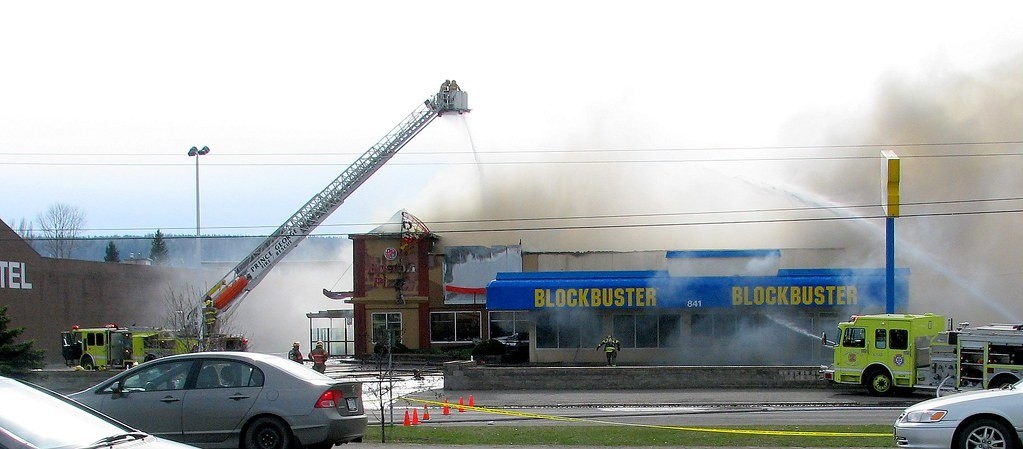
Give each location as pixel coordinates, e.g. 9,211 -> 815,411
817,311 -> 1023,393
60,79 -> 472,377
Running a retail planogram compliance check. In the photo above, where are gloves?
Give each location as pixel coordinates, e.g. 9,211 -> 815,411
596,346 -> 600,350
617,347 -> 621,351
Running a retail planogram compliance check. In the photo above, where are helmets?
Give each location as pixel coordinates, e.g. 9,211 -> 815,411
292,342 -> 300,346
316,340 -> 323,346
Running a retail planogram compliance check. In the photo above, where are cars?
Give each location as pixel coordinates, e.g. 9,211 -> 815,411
0,375 -> 203,449
66,351 -> 367,449
893,378 -> 1023,449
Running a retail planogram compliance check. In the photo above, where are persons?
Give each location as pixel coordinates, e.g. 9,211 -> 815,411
597,336 -> 621,368
288,342 -> 304,364
308,341 -> 328,374
241,338 -> 250,352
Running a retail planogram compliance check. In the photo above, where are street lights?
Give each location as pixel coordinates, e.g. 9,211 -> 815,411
188,145 -> 211,352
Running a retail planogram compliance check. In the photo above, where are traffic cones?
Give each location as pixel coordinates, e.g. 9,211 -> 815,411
458,396 -> 467,412
468,394 -> 474,407
441,399 -> 451,415
410,408 -> 421,425
422,403 -> 430,421
403,408 -> 411,427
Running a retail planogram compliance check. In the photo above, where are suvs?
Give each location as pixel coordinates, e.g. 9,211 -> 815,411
505,332 -> 529,356
